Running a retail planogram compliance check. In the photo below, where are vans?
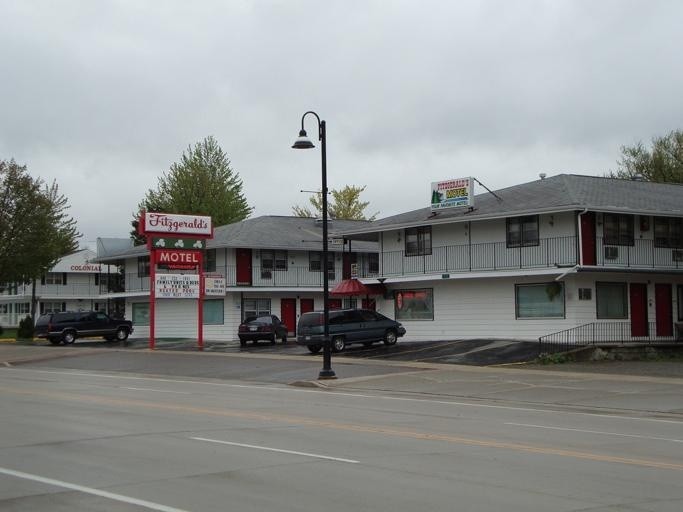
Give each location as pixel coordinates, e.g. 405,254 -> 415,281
297,309 -> 406,352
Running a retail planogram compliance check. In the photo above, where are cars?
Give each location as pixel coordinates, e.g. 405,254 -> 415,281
35,311 -> 134,345
238,315 -> 288,344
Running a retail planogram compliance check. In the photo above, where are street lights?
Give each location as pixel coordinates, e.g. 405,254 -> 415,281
292,112 -> 335,378
